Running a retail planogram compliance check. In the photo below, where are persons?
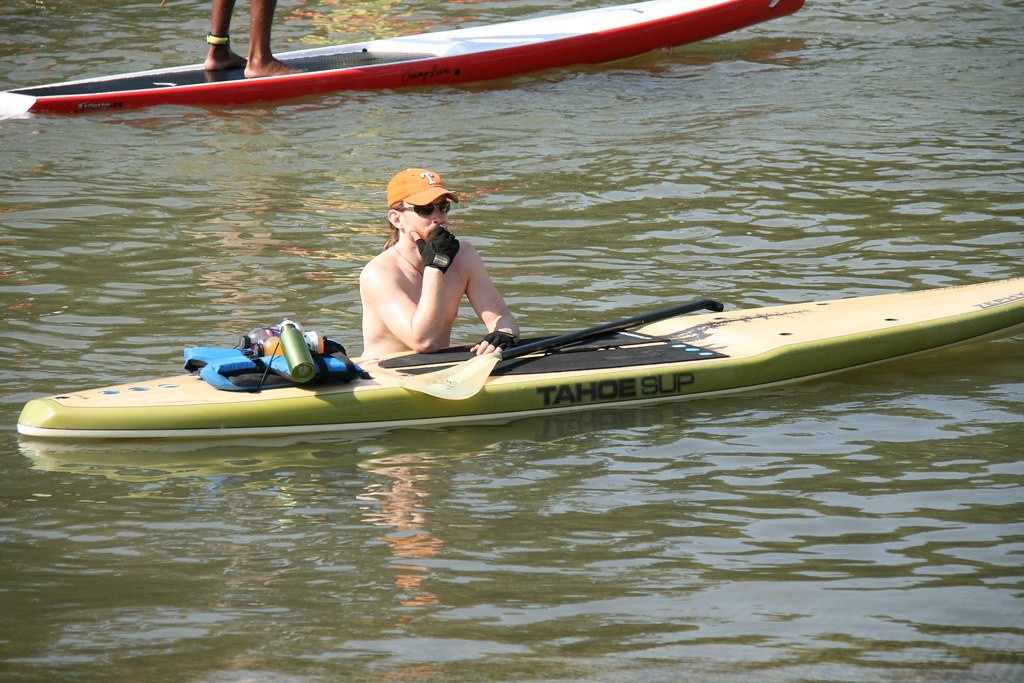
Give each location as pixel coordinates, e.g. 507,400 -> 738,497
360,167 -> 522,361
204,0 -> 310,78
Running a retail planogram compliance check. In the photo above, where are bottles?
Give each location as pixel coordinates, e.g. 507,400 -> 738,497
239,321 -> 304,350
253,332 -> 324,357
280,317 -> 316,383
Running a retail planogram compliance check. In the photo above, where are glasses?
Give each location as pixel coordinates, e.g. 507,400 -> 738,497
413,200 -> 451,215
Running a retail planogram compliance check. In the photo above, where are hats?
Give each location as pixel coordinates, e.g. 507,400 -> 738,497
387,167 -> 459,207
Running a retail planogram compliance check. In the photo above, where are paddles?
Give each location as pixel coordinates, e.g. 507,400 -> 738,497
397,297 -> 724,402
